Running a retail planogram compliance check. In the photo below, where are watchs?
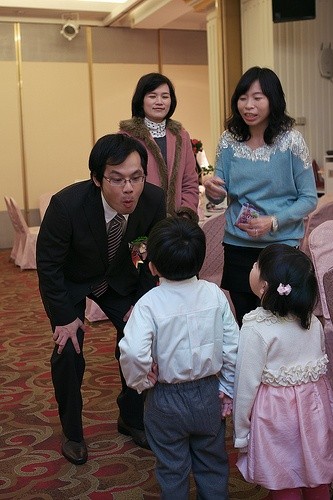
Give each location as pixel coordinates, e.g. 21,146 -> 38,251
270,216 -> 278,234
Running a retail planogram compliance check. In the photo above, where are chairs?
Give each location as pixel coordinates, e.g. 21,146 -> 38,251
85,196 -> 333,385
2,195 -> 43,270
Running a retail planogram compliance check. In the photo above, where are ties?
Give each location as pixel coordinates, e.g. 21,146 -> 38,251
91,215 -> 125,299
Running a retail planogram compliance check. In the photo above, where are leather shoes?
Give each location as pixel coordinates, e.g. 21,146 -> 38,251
61,435 -> 89,466
116,416 -> 153,451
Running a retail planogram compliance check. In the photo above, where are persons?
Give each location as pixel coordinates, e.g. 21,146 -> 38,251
38,134 -> 167,465
118,72 -> 199,229
202,65 -> 318,330
234,243 -> 333,500
117,217 -> 240,500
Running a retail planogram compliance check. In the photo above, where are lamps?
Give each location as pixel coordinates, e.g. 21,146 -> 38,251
59,20 -> 81,41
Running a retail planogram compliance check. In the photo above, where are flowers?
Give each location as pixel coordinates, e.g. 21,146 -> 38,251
190,137 -> 204,155
129,237 -> 148,266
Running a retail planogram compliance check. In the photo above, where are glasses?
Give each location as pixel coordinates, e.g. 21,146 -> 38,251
102,175 -> 147,187
